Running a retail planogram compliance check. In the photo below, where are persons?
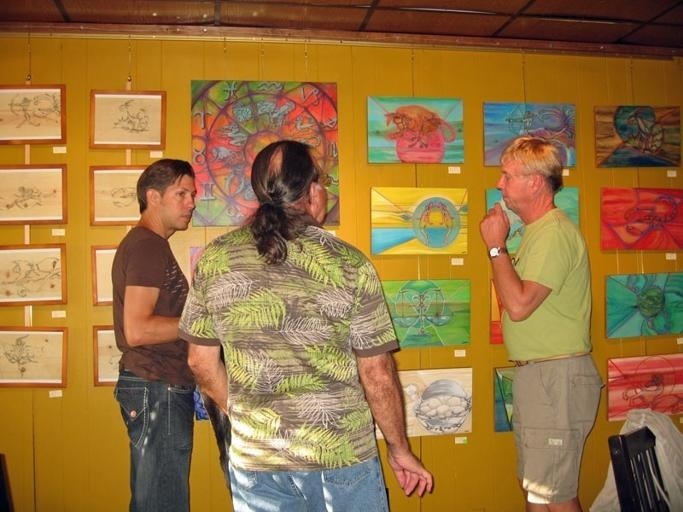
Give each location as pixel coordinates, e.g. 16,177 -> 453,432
111,159 -> 197,512
178,139 -> 434,512
479,134 -> 605,512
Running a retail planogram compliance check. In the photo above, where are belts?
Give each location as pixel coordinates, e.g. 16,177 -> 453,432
509,350 -> 590,366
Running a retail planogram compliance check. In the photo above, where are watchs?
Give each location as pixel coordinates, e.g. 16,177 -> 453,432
487,246 -> 508,258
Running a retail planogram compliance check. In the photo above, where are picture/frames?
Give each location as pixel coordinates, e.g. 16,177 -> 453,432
0,83 -> 68,389
87,89 -> 165,386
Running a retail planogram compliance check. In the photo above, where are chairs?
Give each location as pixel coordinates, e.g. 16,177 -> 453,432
609,423 -> 671,509
